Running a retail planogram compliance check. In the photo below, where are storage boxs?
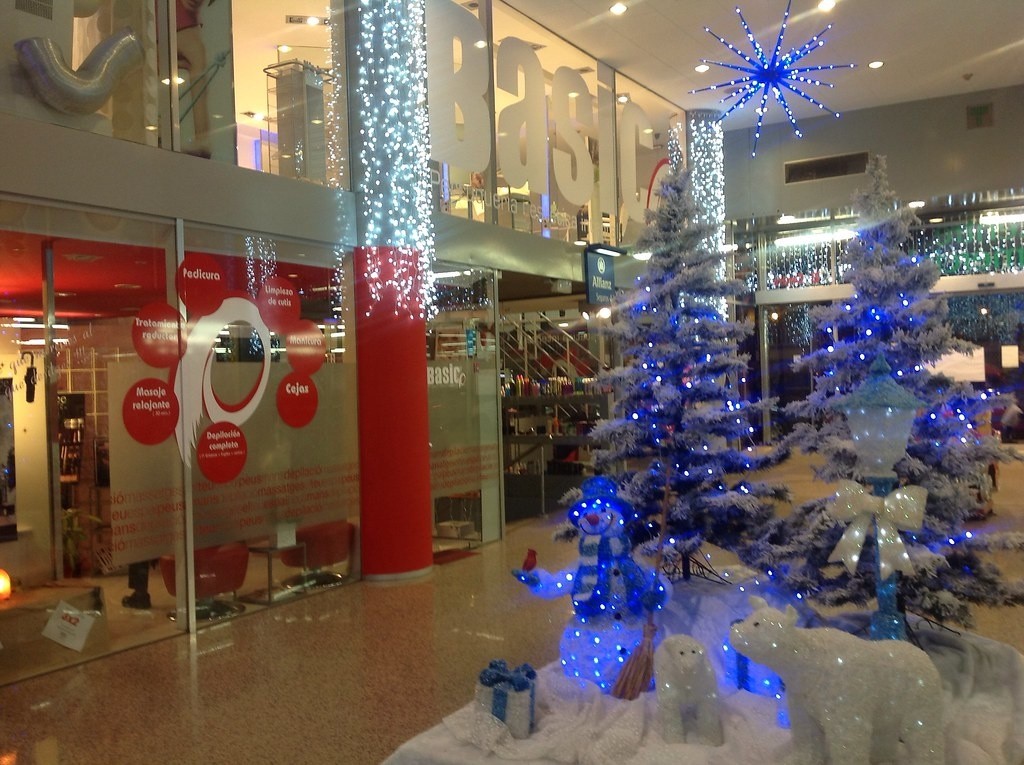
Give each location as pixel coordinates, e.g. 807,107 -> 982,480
437,520 -> 475,537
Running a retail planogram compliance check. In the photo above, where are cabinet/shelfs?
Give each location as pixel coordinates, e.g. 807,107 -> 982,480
502,391 -> 616,500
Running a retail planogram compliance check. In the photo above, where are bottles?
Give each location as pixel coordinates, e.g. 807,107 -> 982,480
515,374 -> 599,395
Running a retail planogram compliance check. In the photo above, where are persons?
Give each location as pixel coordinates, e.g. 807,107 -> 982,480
4,434 -> 16,515
1003,397 -> 1024,442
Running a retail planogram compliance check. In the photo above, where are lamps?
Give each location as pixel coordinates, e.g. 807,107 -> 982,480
589,242 -> 627,257
688,0 -> 860,159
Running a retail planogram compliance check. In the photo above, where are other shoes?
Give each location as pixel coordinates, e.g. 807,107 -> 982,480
123,592 -> 151,610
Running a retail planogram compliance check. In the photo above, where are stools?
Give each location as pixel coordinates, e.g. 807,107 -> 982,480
160,540 -> 249,623
281,520 -> 356,588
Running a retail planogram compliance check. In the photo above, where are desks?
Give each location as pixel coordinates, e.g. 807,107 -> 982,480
232,534 -> 308,607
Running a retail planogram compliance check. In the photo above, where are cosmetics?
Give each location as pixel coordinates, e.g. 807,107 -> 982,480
501,375 -> 612,476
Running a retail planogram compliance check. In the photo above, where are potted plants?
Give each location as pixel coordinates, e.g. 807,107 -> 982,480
62,506 -> 103,578
